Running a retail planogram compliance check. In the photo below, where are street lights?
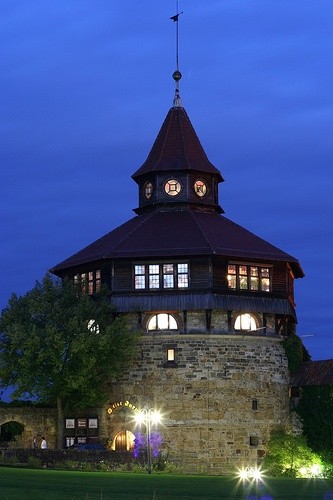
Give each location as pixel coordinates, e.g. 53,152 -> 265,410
136,408 -> 162,474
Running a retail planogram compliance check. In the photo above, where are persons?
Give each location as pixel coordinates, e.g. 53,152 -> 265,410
32,437 -> 37,448
40,436 -> 47,449
